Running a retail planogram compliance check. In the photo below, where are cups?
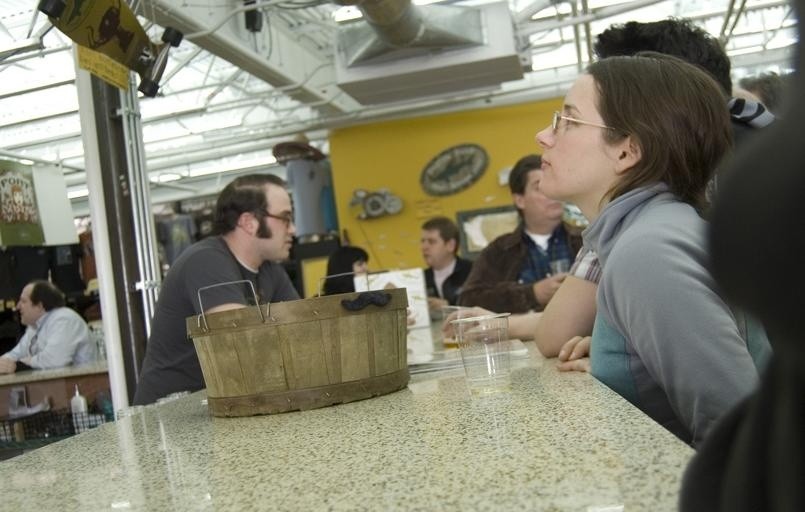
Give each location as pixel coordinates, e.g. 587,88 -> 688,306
448,312 -> 512,380
441,305 -> 473,348
549,258 -> 570,276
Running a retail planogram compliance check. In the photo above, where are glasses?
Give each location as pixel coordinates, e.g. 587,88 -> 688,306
550,109 -> 618,130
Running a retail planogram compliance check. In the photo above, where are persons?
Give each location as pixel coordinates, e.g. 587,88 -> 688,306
1,279 -> 101,441
324,244 -> 369,294
131,173 -> 301,405
418,215 -> 473,306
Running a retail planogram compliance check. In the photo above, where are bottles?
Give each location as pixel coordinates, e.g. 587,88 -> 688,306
71,385 -> 88,433
